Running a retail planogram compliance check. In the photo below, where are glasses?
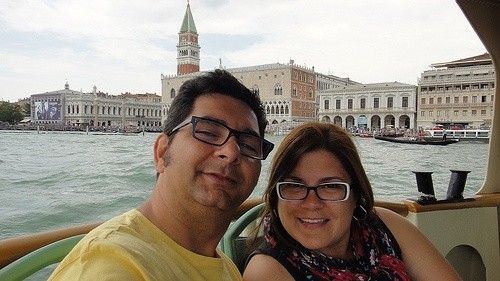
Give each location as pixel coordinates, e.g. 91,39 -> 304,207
166,115 -> 275,160
276,180 -> 350,201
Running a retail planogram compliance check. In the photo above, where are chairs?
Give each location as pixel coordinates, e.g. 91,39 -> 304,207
0,234 -> 85,281
223,203 -> 273,273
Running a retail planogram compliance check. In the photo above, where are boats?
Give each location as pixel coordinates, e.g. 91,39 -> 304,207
374,129 -> 453,146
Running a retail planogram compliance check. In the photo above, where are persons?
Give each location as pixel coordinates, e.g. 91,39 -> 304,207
442,129 -> 446,141
46,70 -> 276,281
348,125 -> 425,136
242,121 -> 463,281
